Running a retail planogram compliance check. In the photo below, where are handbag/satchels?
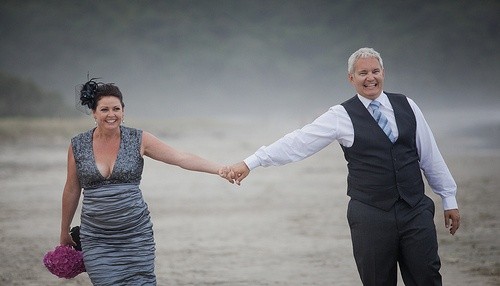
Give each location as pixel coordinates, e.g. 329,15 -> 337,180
70,226 -> 89,252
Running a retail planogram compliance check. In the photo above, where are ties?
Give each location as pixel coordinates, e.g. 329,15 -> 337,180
369,101 -> 397,143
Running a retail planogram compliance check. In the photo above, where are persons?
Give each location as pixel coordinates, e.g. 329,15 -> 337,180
220,48 -> 461,286
60,78 -> 237,286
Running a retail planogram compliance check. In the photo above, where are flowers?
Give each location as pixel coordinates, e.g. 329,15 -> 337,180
44,226 -> 87,278
